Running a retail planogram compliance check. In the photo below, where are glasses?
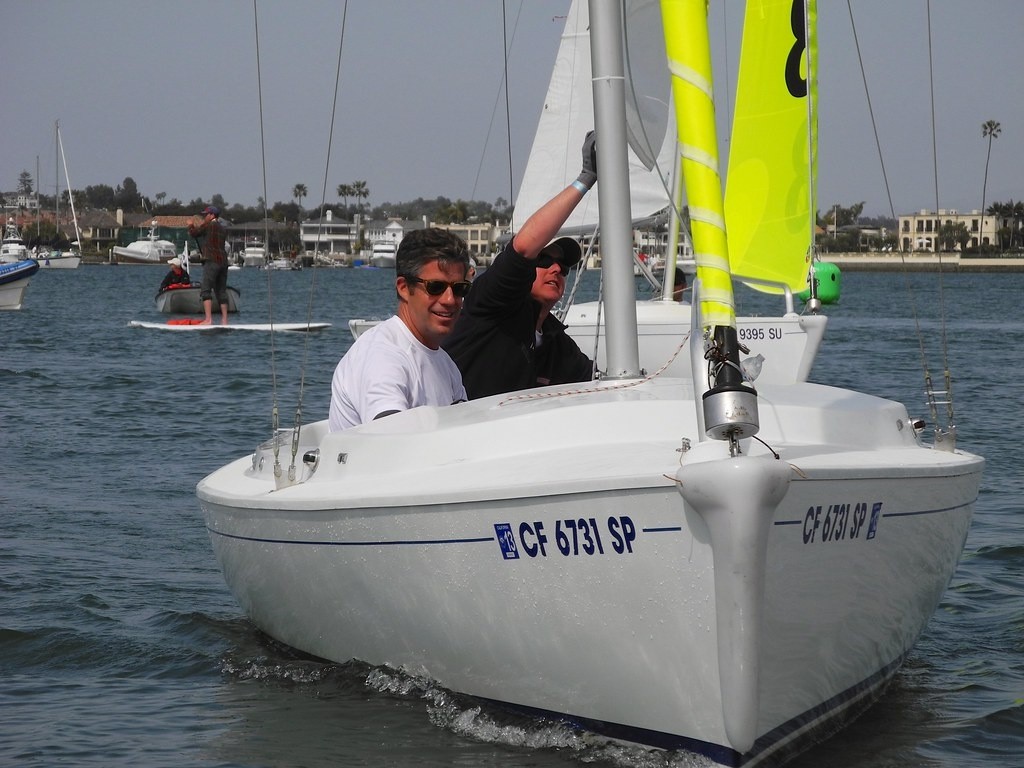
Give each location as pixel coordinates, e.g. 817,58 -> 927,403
404,275 -> 472,297
537,253 -> 572,278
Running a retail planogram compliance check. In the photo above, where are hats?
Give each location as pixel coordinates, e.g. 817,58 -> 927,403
200,206 -> 220,217
167,258 -> 181,265
537,236 -> 582,267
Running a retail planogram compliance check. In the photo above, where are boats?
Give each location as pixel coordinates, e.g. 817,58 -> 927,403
0,258 -> 40,311
111,206 -> 178,266
175,222 -> 302,271
1,204 -> 31,264
368,233 -> 399,269
152,281 -> 242,316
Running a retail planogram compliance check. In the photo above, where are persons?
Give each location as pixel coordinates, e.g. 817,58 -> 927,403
329,225 -> 470,438
186,205 -> 229,327
436,130 -> 599,400
158,258 -> 190,291
661,269 -> 691,305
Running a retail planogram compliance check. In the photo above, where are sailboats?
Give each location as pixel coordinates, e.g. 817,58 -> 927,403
345,0 -> 843,383
30,117 -> 84,269
187,0 -> 993,767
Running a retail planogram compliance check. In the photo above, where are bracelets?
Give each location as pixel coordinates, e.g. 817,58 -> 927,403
572,181 -> 589,196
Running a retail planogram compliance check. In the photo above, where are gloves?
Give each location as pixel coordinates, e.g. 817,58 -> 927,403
576,130 -> 597,189
